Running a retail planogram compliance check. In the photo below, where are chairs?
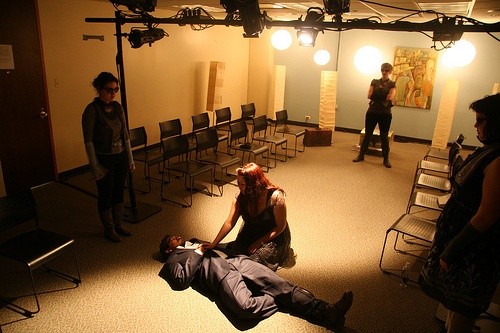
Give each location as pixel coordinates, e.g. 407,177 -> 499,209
127,102 -> 306,209
379,134 -> 465,285
0,188 -> 81,317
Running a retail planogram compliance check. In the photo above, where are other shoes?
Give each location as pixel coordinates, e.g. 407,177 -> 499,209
284,248 -> 295,268
323,290 -> 354,332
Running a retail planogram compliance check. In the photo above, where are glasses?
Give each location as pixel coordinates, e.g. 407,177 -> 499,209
103,87 -> 119,94
381,69 -> 389,72
476,117 -> 487,124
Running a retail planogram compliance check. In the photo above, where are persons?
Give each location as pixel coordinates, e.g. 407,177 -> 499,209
202,162 -> 291,272
352,63 -> 398,168
81,72 -> 136,243
161,235 -> 354,331
418,92 -> 500,333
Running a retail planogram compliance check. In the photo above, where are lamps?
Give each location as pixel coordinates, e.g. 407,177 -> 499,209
106,0 -> 464,48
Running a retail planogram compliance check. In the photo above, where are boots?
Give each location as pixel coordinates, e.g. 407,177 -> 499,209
352,140 -> 368,162
381,148 -> 392,168
98,208 -> 121,243
113,203 -> 133,236
